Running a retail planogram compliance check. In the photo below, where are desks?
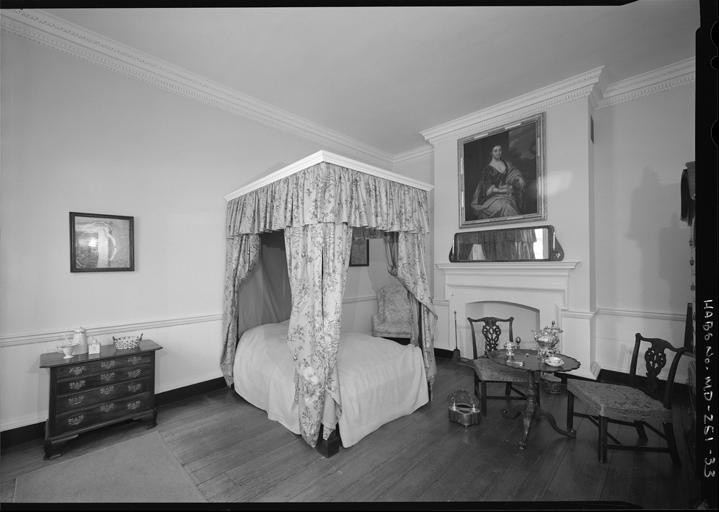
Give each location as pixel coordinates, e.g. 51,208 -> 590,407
488,348 -> 580,451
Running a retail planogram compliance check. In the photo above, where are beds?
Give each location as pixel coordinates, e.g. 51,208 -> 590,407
236,318 -> 426,450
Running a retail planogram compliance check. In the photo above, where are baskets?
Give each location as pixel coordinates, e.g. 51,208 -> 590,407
111,333 -> 144,349
447,388 -> 480,427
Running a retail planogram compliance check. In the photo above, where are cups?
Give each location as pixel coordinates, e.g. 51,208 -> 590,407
57,344 -> 79,360
546,356 -> 561,366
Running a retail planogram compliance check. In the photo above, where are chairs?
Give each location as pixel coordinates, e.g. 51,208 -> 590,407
566,331 -> 686,469
372,283 -> 418,346
467,315 -> 540,417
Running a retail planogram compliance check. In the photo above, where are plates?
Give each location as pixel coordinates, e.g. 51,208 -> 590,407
543,359 -> 565,367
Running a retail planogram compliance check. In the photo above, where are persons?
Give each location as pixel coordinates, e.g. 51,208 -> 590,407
471,144 -> 527,219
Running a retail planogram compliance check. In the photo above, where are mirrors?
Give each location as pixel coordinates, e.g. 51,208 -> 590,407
454,227 -> 554,262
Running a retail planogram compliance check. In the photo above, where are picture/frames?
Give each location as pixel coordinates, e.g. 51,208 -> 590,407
350,238 -> 369,267
457,112 -> 547,228
69,211 -> 134,274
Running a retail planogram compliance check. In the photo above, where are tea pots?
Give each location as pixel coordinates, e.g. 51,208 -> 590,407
69,327 -> 90,355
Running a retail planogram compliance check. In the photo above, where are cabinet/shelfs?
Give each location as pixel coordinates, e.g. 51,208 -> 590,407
39,339 -> 162,460
